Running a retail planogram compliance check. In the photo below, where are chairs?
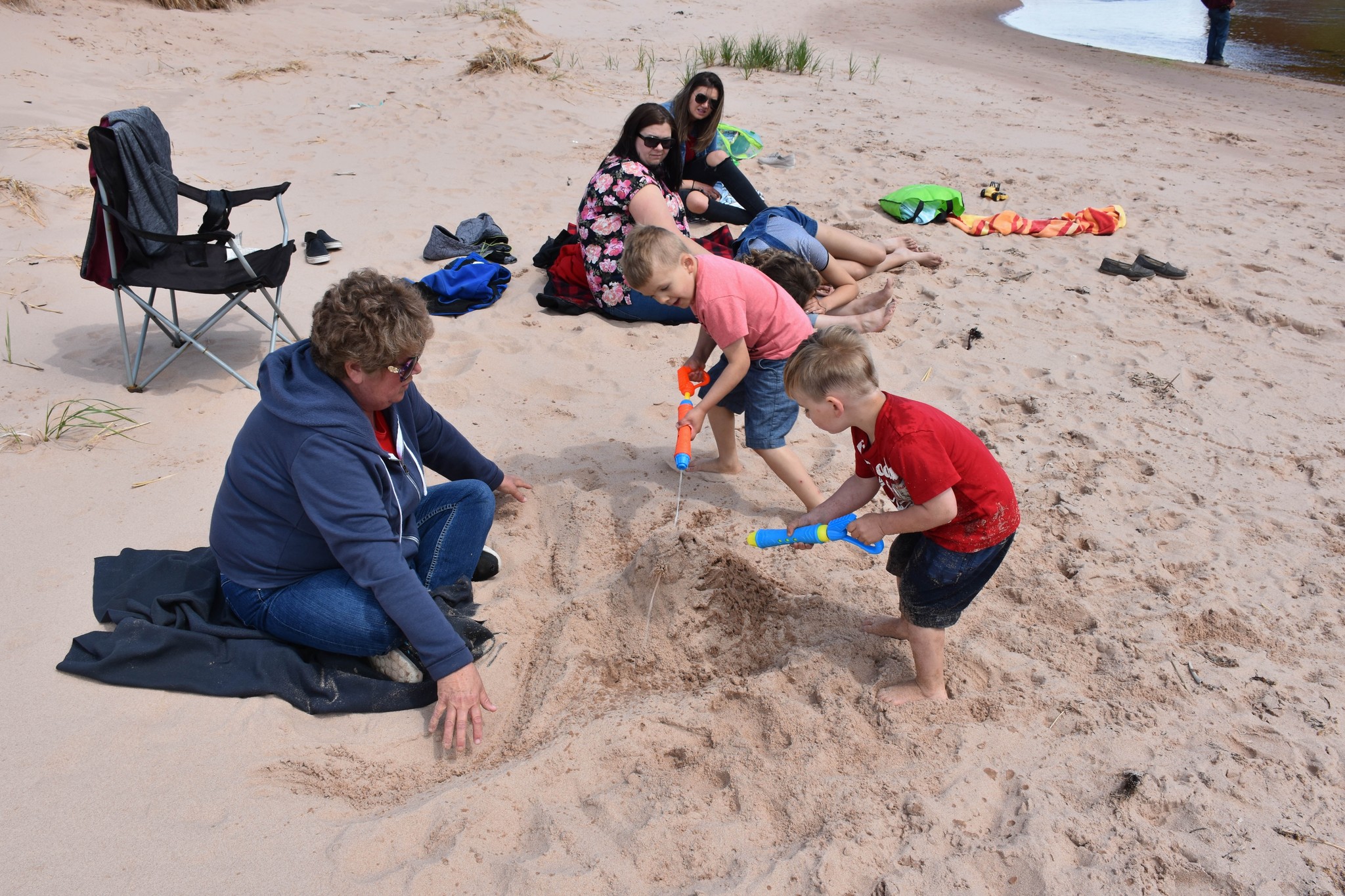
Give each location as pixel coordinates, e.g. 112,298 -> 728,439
79,106 -> 303,392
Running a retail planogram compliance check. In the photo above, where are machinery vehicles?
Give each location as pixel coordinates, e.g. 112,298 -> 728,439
980,181 -> 1008,201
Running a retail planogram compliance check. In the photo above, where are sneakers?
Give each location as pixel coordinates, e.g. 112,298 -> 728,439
306,238 -> 330,264
470,545 -> 502,583
758,152 -> 796,170
369,639 -> 429,683
304,230 -> 342,249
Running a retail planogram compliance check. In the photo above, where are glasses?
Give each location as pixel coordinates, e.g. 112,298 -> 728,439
636,131 -> 677,150
380,353 -> 421,383
692,90 -> 720,111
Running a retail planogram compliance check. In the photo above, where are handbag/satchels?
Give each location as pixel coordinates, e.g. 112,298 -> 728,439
878,184 -> 965,224
707,120 -> 764,165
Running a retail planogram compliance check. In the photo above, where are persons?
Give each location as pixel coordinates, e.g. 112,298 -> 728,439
660,72 -> 768,225
621,227 -> 828,512
783,325 -> 1021,706
578,102 -> 896,332
736,206 -> 944,313
1201,0 -> 1237,66
208,267 -> 535,753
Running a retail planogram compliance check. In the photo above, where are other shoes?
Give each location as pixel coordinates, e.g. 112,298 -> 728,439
1205,58 -> 1230,68
1134,254 -> 1187,279
1098,257 -> 1156,281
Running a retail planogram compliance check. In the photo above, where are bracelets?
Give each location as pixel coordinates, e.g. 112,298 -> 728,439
692,180 -> 695,189
